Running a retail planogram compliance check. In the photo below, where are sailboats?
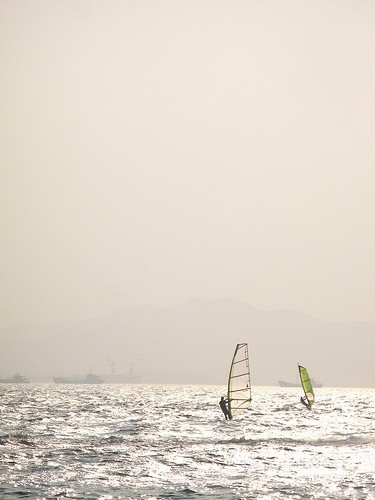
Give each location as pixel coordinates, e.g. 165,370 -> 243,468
298,365 -> 315,410
228,343 -> 252,420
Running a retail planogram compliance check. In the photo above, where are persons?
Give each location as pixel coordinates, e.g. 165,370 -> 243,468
300,396 -> 310,409
219,397 -> 233,421
